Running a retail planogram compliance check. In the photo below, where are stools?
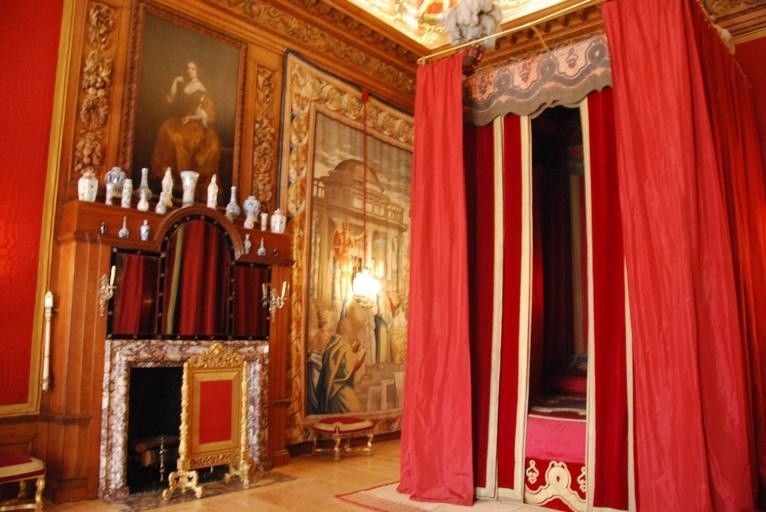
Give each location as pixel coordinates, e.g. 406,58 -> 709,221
1,452 -> 49,512
310,413 -> 374,460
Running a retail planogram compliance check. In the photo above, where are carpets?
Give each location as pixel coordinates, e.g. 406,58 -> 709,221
334,479 -> 565,512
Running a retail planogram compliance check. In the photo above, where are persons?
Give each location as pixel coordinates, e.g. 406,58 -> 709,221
150,59 -> 224,204
308,217 -> 401,413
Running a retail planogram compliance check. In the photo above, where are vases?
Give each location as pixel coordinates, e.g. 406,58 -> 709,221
74,164 -> 290,256
